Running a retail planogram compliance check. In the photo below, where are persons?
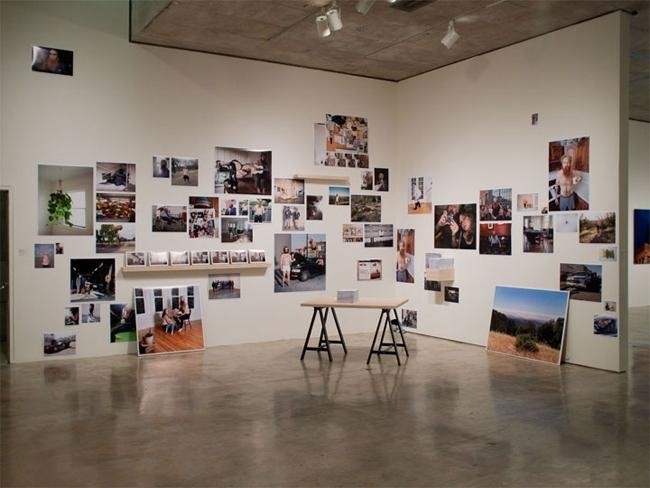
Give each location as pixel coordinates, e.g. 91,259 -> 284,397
292,206 -> 301,229
88,303 -> 97,322
111,303 -> 135,341
177,294 -> 191,332
437,204 -> 462,248
37,49 -> 72,73
487,228 -> 502,248
284,206 -> 291,229
541,227 -> 550,249
412,181 -> 423,211
554,153 -> 583,210
397,240 -> 412,282
334,192 -> 341,204
75,272 -> 84,292
40,251 -> 50,268
279,244 -> 297,288
378,227 -> 386,241
151,156 -> 267,243
458,205 -> 475,247
161,307 -> 176,335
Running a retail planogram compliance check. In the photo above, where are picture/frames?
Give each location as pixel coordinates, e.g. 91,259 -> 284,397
485,285 -> 570,366
124,248 -> 266,267
133,284 -> 205,357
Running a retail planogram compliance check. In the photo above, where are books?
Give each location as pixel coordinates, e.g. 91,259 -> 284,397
335,286 -> 361,302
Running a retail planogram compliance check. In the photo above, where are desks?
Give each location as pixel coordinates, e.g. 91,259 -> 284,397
299,295 -> 410,366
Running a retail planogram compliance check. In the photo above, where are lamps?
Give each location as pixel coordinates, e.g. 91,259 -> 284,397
312,0 -> 462,50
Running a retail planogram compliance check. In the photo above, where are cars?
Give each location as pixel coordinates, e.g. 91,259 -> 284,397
44,335 -> 72,354
286,250 -> 326,282
565,270 -> 592,291
593,311 -> 618,336
217,279 -> 230,288
